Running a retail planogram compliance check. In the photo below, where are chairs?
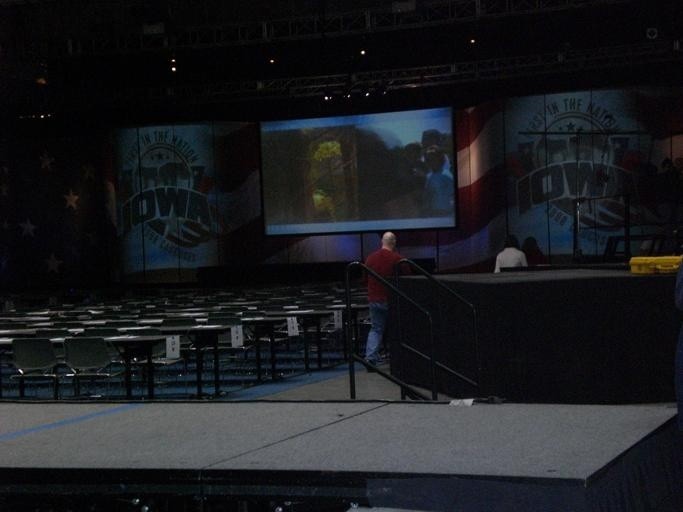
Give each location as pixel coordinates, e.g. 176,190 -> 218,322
0,282 -> 382,372
1,316 -> 306,402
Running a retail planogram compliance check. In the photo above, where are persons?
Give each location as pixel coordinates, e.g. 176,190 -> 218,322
368,129 -> 454,218
362,232 -> 411,372
521,237 -> 550,270
494,236 -> 528,273
